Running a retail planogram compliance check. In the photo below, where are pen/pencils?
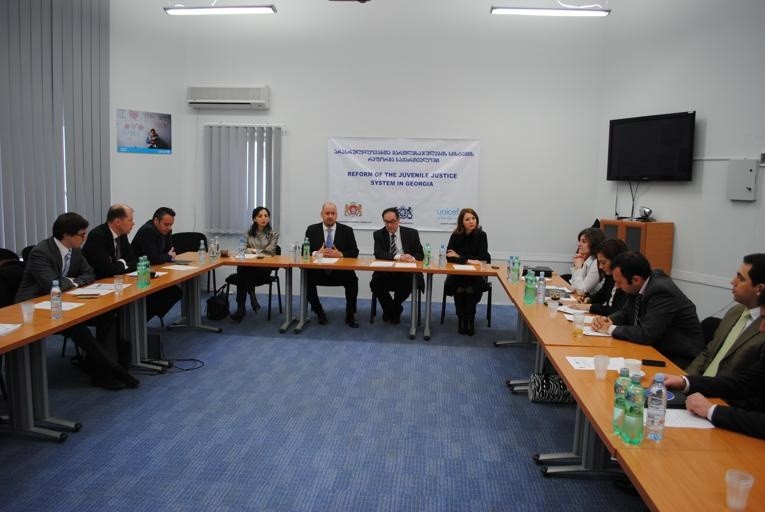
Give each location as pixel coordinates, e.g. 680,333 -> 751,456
581,289 -> 586,304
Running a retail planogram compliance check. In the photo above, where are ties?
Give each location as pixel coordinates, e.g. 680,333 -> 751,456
115,238 -> 120,258
391,235 -> 396,255
326,229 -> 333,247
633,296 -> 642,327
703,312 -> 750,377
61,253 -> 70,276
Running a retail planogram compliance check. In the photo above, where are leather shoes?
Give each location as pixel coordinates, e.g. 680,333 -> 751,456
108,365 -> 139,387
382,312 -> 400,324
345,318 -> 358,328
90,373 -> 125,389
317,314 -> 327,325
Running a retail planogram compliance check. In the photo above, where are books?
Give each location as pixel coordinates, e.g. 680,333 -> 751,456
164,260 -> 193,265
643,387 -> 687,409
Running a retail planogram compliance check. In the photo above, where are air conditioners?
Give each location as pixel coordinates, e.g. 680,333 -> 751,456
184,80 -> 272,112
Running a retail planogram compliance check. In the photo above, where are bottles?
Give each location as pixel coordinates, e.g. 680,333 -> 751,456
51,280 -> 63,320
523,269 -> 546,304
137,256 -> 150,289
506,255 -> 520,285
613,368 -> 667,446
295,237 -> 310,262
423,242 -> 446,268
199,236 -> 220,266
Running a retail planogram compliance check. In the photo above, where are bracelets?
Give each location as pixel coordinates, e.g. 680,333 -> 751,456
680,376 -> 687,388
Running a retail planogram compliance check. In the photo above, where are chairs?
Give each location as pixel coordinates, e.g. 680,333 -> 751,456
0,249 -> 33,310
370,276 -> 422,329
677,314 -> 720,370
170,231 -> 218,299
441,254 -> 492,326
226,245 -> 281,318
19,244 -> 102,357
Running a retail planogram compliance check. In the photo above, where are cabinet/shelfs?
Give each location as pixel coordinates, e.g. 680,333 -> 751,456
599,217 -> 676,278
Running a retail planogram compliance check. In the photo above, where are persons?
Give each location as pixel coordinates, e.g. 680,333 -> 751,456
657,287 -> 765,441
445,208 -> 491,335
16,212 -> 140,388
569,238 -> 630,315
301,202 -> 359,327
130,207 -> 184,322
370,209 -> 424,324
144,128 -> 160,148
82,203 -> 137,280
229,206 -> 279,323
570,228 -> 606,296
592,250 -> 705,370
685,253 -> 765,375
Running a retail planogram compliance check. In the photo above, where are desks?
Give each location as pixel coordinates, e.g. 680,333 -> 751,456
1,317 -> 68,442
494,264 -> 571,346
542,346 -> 764,473
513,302 -> 656,464
174,250 -> 293,334
2,288 -> 162,433
83,262 -> 226,365
620,451 -> 764,512
421,261 -> 496,343
291,255 -> 423,340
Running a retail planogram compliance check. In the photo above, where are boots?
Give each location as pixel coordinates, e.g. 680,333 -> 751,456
458,312 -> 474,336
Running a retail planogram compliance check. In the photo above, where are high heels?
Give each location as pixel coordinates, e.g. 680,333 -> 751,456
250,298 -> 261,313
231,311 -> 245,323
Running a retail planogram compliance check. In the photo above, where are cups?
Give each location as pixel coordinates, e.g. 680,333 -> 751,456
594,355 -> 609,380
480,261 -> 487,272
550,290 -> 563,303
114,275 -> 124,296
316,252 -> 324,262
572,313 -> 584,340
220,247 -> 229,258
21,302 -> 34,322
725,470 -> 754,509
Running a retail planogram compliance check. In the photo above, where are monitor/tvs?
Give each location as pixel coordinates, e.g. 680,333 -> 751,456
607,111 -> 696,181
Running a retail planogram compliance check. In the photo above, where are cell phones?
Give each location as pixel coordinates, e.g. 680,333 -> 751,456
76,294 -> 100,299
643,360 -> 665,367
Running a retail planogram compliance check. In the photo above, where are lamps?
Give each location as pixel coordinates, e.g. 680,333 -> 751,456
162,5 -> 280,19
488,5 -> 615,19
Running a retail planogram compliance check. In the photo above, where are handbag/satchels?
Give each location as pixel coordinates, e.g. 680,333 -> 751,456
529,372 -> 574,405
207,285 -> 229,320
